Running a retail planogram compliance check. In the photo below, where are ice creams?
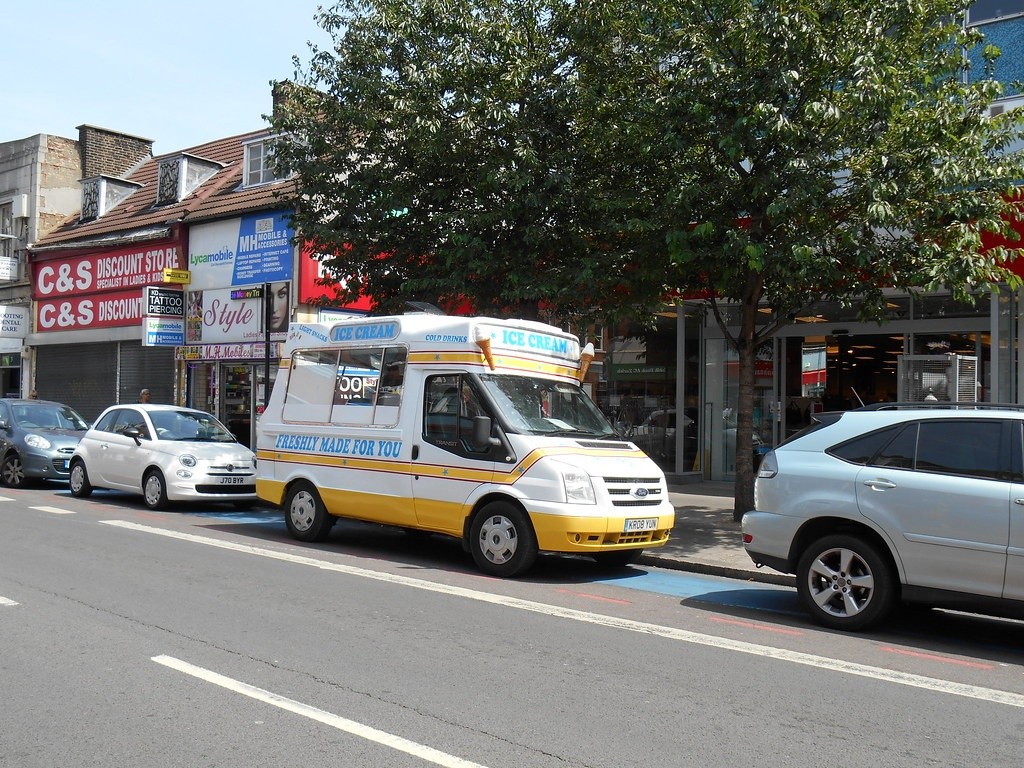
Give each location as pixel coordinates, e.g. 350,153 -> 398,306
474,325 -> 495,371
578,342 -> 594,381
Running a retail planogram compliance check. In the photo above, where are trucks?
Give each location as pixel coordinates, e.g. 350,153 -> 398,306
255,311 -> 677,578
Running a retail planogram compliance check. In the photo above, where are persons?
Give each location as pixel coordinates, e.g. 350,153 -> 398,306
133,389 -> 152,404
27,390 -> 37,399
270,282 -> 289,332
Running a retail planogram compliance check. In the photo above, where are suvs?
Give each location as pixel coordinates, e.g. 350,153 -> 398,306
741,401 -> 1024,633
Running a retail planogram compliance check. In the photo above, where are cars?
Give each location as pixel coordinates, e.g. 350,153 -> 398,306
666,416 -> 765,463
68,403 -> 257,510
0,398 -> 93,489
596,404 -> 700,446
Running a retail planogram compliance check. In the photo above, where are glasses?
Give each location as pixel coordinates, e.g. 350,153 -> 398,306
144,392 -> 151,395
